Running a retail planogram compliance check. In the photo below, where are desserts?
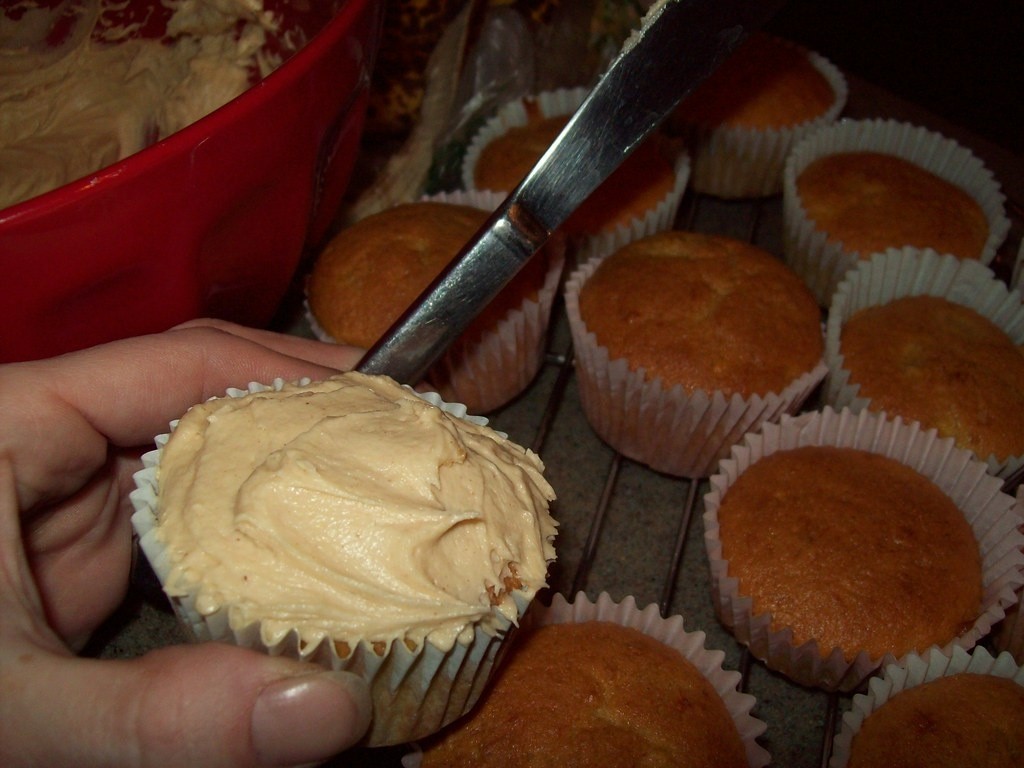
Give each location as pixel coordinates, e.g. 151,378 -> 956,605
127,27 -> 1023,768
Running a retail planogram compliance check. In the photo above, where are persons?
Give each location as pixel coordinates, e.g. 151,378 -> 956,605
0,313 -> 440,768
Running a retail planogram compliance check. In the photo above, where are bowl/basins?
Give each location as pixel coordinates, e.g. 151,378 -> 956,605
0,2 -> 375,374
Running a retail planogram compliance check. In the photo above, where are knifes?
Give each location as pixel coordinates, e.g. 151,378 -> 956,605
316,2 -> 763,387
